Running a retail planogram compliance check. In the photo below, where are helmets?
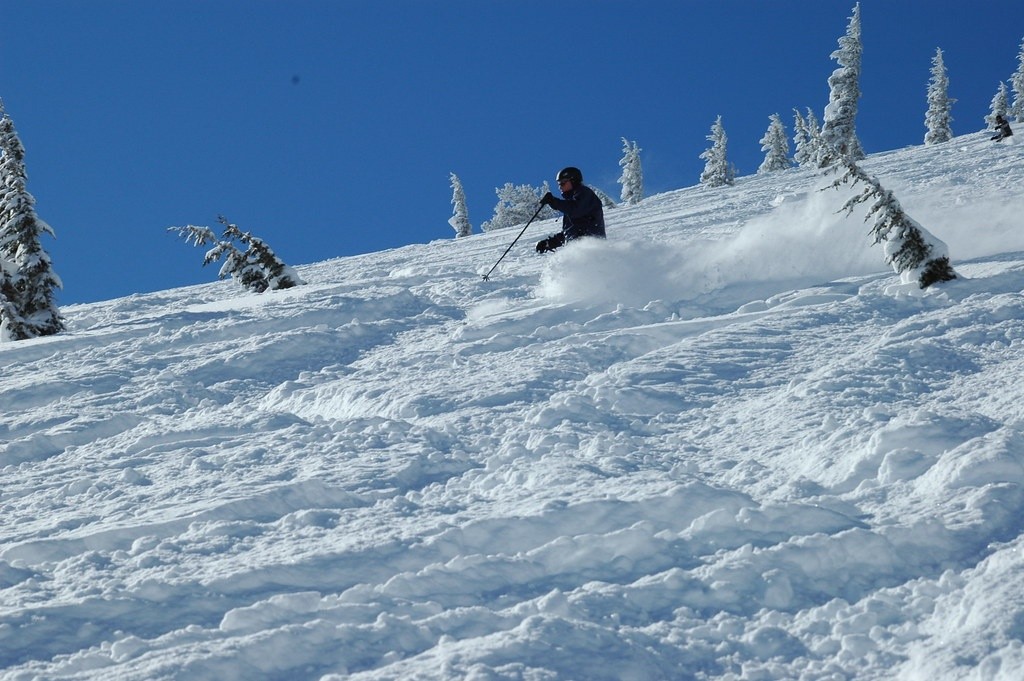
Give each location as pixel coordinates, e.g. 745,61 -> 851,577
996,116 -> 1002,125
556,167 -> 583,198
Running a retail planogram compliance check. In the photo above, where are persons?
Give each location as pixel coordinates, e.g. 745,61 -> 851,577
991,116 -> 1012,142
536,167 -> 606,253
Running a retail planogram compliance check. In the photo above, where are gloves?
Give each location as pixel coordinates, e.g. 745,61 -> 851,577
536,232 -> 565,252
995,126 -> 1001,130
539,192 -> 559,210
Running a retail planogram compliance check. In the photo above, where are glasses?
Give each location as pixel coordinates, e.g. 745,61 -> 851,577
559,181 -> 568,186
